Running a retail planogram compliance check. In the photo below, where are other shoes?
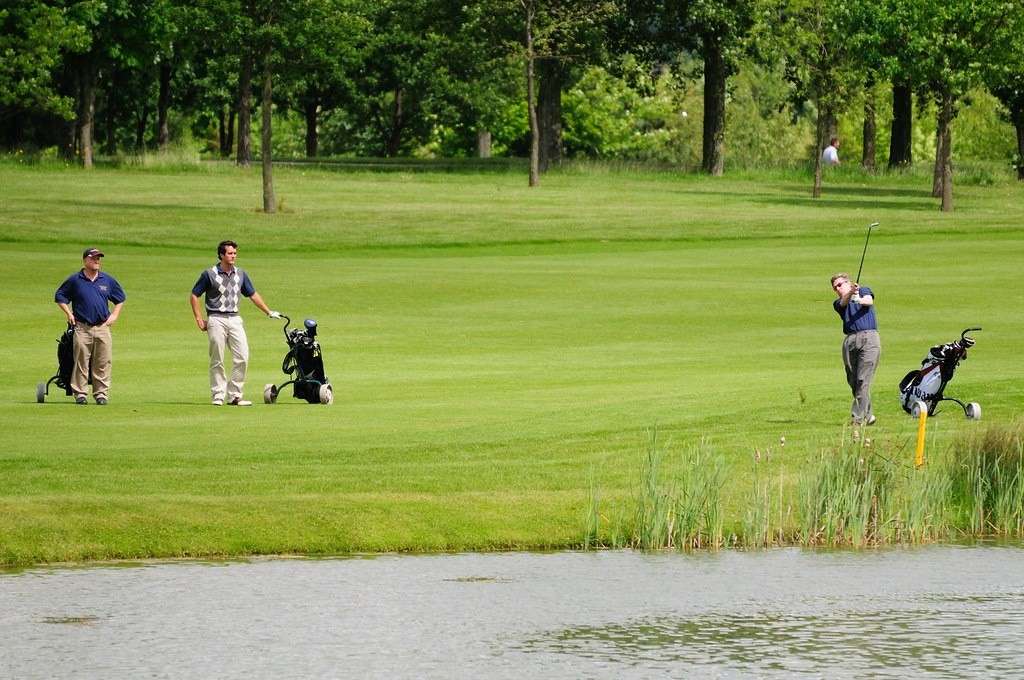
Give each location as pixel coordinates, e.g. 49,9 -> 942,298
849,422 -> 861,428
227,397 -> 252,406
97,398 -> 107,405
76,397 -> 87,404
212,398 -> 223,406
867,415 -> 876,426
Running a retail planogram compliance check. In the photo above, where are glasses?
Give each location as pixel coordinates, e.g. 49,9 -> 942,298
834,281 -> 847,291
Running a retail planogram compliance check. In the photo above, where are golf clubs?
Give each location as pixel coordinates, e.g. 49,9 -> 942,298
938,336 -> 975,357
851,222 -> 879,303
290,318 -> 322,354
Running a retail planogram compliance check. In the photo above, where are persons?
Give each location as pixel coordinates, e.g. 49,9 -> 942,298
831,272 -> 881,428
54,248 -> 125,405
189,240 -> 282,406
822,137 -> 841,164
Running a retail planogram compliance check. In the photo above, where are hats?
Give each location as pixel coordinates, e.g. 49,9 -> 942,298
83,248 -> 104,258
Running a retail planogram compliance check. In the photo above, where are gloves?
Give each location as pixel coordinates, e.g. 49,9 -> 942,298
851,292 -> 859,305
268,311 -> 281,319
